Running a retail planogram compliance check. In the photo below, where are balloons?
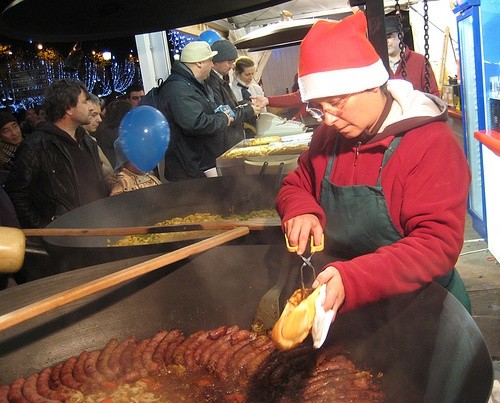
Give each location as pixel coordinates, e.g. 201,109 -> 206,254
198,29 -> 222,48
119,106 -> 171,175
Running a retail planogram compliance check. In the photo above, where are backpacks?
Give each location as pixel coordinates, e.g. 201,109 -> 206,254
138,74 -> 207,170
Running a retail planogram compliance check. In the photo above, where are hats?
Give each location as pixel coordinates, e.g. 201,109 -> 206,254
384,16 -> 410,36
0,111 -> 19,130
211,40 -> 239,63
113,138 -> 128,172
297,9 -> 390,104
180,41 -> 219,63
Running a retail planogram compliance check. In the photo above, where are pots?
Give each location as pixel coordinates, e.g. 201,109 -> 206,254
0,244 -> 493,403
40,174 -> 293,274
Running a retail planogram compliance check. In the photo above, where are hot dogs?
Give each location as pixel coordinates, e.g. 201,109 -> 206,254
271,287 -> 318,347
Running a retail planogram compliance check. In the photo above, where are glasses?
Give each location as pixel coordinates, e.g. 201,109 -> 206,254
305,90 -> 352,120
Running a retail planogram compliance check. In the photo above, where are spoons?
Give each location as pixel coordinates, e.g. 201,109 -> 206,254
234,161 -> 269,215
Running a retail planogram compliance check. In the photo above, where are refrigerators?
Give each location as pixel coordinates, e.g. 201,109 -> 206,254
452,0 -> 500,244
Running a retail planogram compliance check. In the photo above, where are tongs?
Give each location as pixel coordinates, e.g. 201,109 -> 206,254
281,229 -> 326,300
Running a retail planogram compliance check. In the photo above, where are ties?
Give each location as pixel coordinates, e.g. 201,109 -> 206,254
237,82 -> 252,100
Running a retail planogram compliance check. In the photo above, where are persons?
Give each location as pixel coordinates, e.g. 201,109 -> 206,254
127,87 -> 145,106
229,57 -> 269,113
107,138 -> 162,194
384,16 -> 439,98
250,89 -> 310,121
157,42 -> 264,180
0,79 -> 116,227
276,10 -> 471,323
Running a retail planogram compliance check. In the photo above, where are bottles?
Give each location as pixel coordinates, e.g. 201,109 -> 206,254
486,76 -> 500,138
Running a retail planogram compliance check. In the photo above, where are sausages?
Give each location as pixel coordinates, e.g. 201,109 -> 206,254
0,326 -> 270,403
271,347 -> 386,403
